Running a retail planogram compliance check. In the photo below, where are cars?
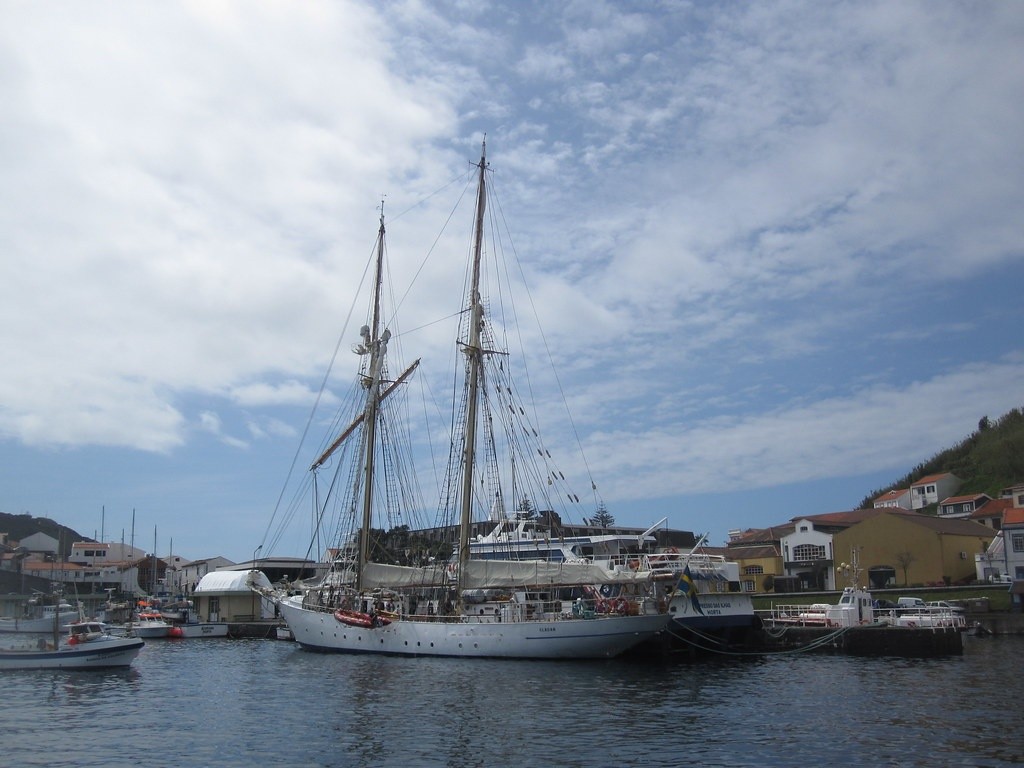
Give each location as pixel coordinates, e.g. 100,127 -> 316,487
871,599 -> 895,609
928,600 -> 962,612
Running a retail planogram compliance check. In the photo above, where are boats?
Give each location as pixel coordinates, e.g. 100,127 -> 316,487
0,572 -> 145,671
333,608 -> 393,629
0,531 -> 79,634
777,547 -> 965,628
444,455 -> 761,657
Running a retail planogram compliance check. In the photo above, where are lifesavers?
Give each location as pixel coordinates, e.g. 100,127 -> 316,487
597,599 -> 613,614
613,597 -> 629,613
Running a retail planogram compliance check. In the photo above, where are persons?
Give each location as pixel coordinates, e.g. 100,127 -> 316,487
373,598 -> 385,610
386,599 -> 394,612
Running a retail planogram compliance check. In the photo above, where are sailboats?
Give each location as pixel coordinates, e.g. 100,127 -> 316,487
88,505 -> 229,640
245,129 -> 725,661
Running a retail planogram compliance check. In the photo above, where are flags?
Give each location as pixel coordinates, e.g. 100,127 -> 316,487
677,566 -> 705,616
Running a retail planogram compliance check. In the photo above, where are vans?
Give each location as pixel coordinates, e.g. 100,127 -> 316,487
898,596 -> 926,607
